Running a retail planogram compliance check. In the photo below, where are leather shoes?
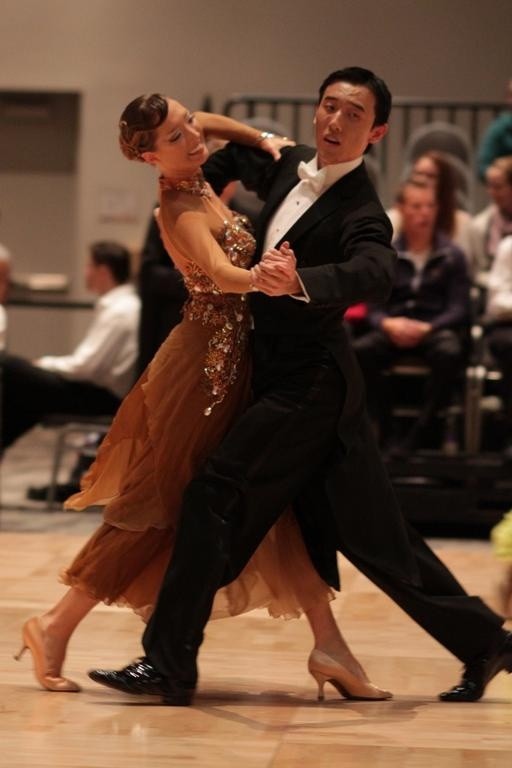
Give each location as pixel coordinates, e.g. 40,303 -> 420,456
88,658 -> 197,705
439,630 -> 511,702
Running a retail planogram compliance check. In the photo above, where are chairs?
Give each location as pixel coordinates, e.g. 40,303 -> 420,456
393,283 -> 512,468
48,414 -> 111,506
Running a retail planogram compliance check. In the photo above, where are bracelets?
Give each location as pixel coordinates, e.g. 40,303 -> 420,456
253,132 -> 271,146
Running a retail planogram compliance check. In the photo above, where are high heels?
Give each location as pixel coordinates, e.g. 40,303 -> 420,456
15,617 -> 82,691
309,648 -> 393,700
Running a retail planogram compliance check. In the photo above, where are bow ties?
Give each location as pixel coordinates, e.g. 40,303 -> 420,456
297,161 -> 327,195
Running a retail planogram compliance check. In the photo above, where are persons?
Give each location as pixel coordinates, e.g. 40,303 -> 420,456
14,93 -> 393,701
88,66 -> 511,706
341,109 -> 512,473
0,238 -> 144,462
